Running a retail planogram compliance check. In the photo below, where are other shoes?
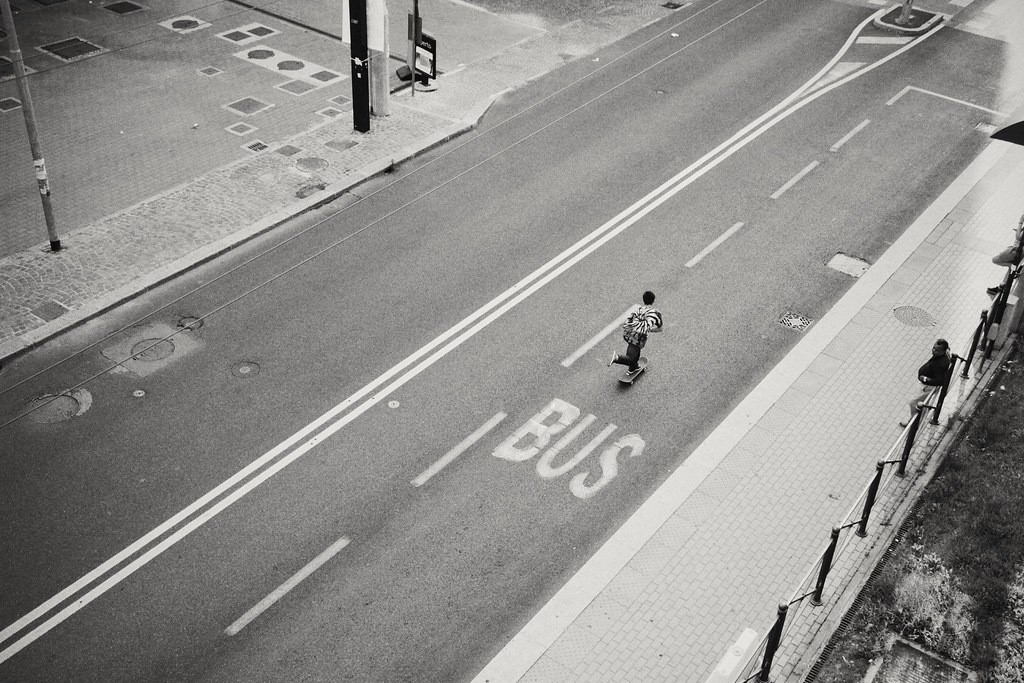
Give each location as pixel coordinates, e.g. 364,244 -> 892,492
627,365 -> 642,374
987,285 -> 1000,295
899,420 -> 909,427
607,350 -> 617,366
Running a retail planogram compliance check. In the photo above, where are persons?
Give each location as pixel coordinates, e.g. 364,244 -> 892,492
606,291 -> 663,375
900,339 -> 951,427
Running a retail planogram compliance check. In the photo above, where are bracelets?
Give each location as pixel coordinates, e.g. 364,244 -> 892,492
924,376 -> 927,382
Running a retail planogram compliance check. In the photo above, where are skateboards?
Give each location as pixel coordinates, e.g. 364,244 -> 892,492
619,356 -> 648,386
